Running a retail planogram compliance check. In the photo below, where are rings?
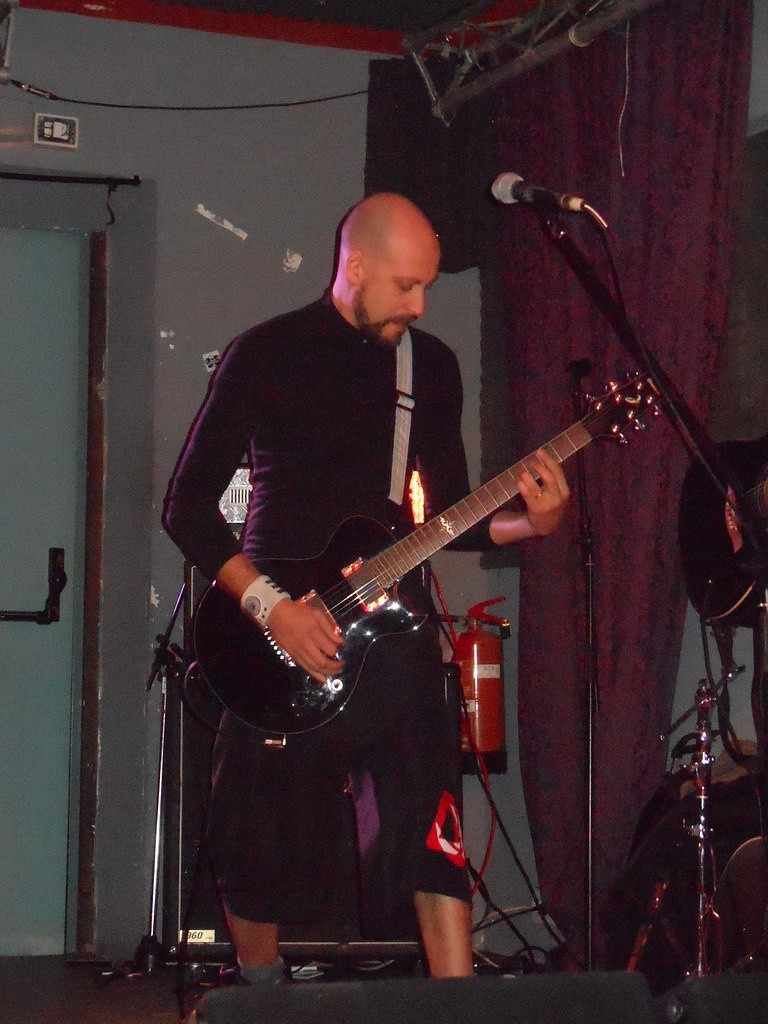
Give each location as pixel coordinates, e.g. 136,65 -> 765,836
530,489 -> 547,498
306,662 -> 317,669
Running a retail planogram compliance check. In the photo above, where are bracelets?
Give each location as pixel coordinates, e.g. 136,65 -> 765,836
240,574 -> 299,669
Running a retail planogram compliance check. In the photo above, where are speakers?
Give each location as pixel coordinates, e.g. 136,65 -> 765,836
183,970 -> 768,1024
166,666 -> 431,975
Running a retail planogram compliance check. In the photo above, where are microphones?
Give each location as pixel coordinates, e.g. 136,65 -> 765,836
491,172 -> 586,211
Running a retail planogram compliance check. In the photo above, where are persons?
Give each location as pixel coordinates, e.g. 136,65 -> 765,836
156,188 -> 574,984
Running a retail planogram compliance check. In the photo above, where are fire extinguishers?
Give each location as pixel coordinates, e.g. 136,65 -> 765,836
437,595 -> 510,753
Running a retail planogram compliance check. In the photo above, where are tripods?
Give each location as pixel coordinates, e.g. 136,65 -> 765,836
92,579 -> 187,993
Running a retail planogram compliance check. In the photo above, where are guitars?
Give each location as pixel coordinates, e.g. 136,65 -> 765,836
676,433 -> 767,632
188,355 -> 665,740
